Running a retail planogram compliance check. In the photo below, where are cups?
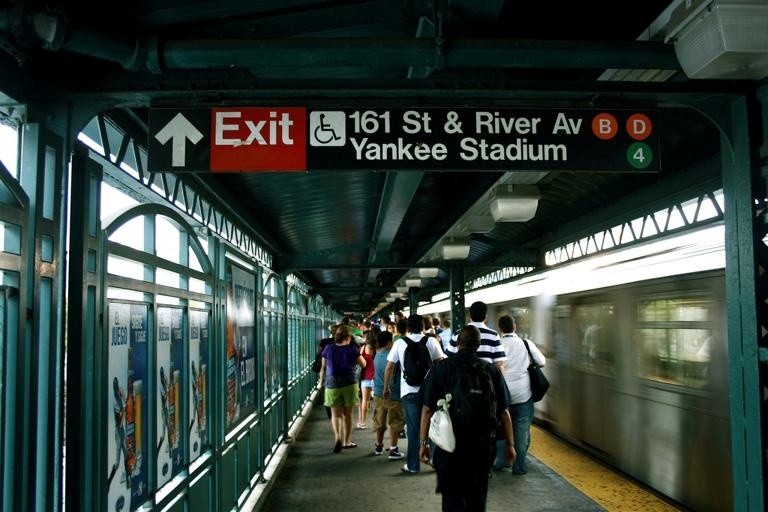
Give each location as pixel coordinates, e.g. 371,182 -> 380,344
170,370 -> 179,433
131,381 -> 144,462
201,364 -> 206,419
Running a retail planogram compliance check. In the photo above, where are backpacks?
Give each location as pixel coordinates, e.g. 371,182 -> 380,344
440,354 -> 501,453
400,334 -> 433,389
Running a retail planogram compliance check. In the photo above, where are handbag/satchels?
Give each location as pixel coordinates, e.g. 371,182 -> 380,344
521,336 -> 552,403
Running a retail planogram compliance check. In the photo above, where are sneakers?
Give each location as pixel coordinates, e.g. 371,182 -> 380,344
398,429 -> 407,439
402,461 -> 421,474
373,440 -> 385,455
356,422 -> 369,430
386,445 -> 406,461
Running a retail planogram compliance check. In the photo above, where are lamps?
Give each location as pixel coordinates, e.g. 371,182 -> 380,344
487,182 -> 541,224
361,263 -> 440,323
440,235 -> 471,260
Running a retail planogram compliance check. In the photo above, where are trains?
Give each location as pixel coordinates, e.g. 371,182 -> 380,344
423,203 -> 731,512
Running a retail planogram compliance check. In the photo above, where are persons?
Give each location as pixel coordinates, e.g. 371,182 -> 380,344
313,302 -> 543,511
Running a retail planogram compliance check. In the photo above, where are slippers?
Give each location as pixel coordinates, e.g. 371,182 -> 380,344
342,440 -> 357,449
332,439 -> 343,454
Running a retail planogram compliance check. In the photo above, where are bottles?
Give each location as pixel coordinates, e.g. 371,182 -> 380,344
228,267 -> 241,429
168,363 -> 176,446
123,376 -> 137,460
197,361 -> 205,419
